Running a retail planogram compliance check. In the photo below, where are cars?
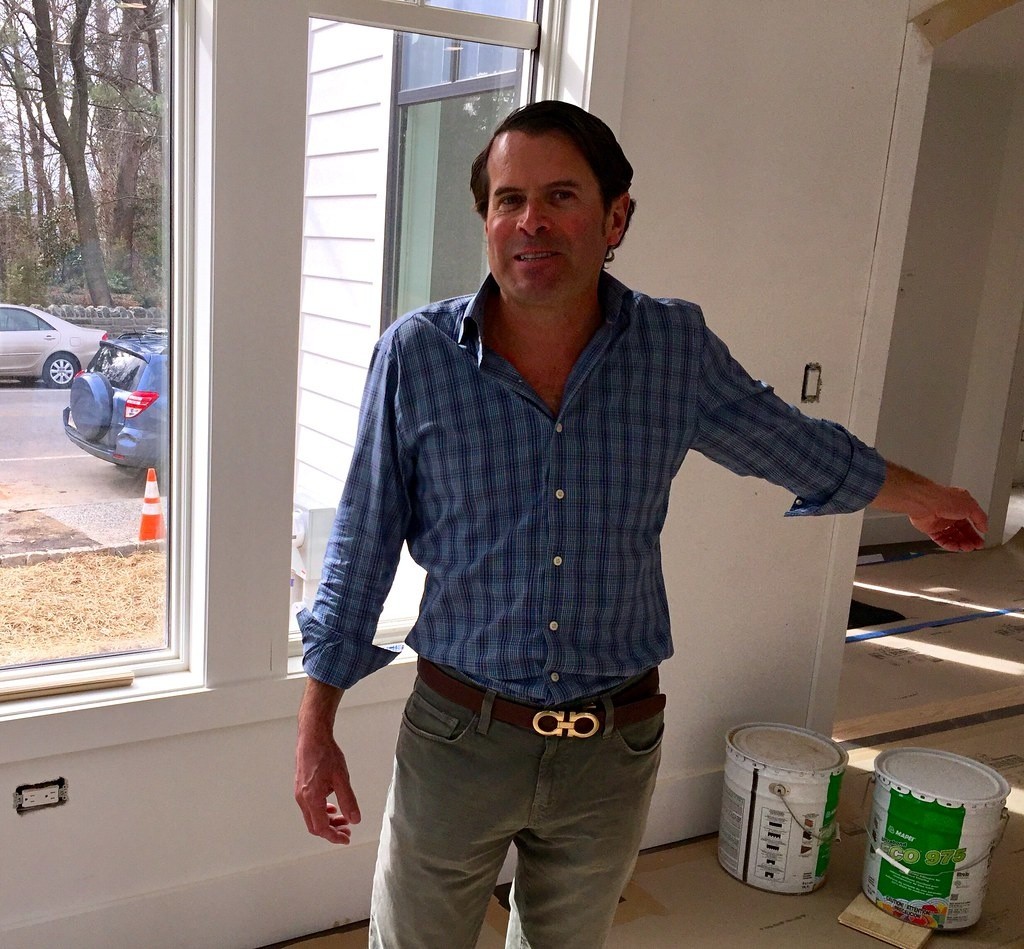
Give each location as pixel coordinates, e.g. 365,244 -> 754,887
0,304 -> 108,390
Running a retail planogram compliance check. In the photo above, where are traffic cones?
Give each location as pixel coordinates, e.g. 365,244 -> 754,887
138,467 -> 168,542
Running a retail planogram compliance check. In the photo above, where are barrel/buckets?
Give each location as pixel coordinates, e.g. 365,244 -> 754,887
718,721 -> 849,893
861,747 -> 1011,929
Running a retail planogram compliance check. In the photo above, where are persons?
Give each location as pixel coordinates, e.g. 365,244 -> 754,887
294,101 -> 989,949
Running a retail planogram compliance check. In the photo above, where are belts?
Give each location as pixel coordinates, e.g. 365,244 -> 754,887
417,654 -> 666,738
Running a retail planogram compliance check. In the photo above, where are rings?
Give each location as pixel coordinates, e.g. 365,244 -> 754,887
944,525 -> 954,530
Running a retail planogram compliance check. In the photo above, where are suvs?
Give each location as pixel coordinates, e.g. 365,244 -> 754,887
62,329 -> 168,471
